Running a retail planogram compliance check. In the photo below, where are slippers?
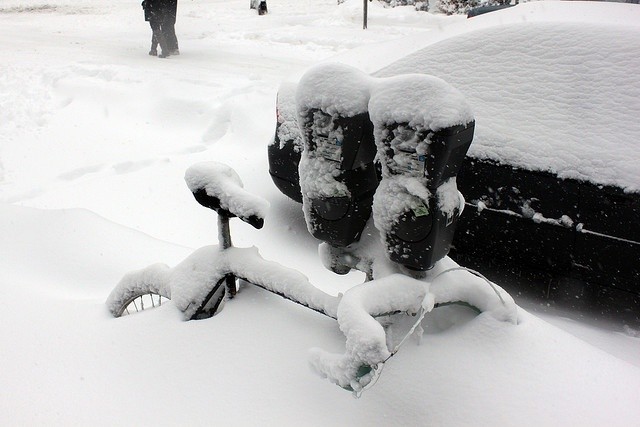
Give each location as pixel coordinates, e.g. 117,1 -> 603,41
156,31 -> 170,58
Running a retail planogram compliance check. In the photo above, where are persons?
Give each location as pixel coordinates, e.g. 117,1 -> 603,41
164,0 -> 180,56
141,0 -> 170,58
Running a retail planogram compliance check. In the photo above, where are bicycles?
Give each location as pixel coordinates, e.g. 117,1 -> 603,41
105,162 -> 523,397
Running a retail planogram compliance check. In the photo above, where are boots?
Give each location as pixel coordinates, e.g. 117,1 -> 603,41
149,32 -> 157,55
